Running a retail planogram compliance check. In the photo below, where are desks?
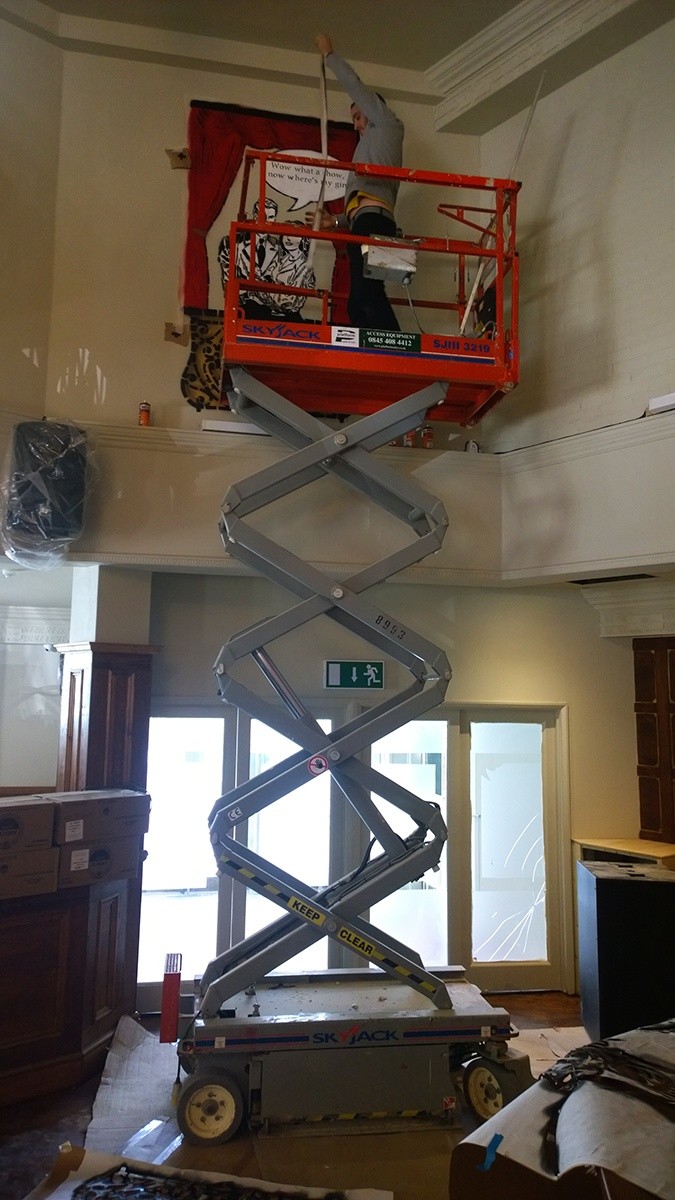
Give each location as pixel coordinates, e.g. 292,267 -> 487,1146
571,838 -> 675,870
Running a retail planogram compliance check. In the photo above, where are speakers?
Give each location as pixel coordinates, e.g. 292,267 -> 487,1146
8,421 -> 88,536
577,861 -> 675,1043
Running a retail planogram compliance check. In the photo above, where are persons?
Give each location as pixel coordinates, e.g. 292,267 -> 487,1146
314,31 -> 404,332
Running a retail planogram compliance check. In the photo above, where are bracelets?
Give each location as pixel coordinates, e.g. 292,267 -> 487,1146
325,51 -> 333,59
330,213 -> 338,231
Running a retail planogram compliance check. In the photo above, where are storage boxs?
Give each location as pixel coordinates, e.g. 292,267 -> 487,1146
0,787 -> 149,899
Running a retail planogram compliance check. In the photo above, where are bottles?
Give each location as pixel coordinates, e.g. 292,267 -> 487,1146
421,424 -> 433,448
404,430 -> 416,448
138,400 -> 151,425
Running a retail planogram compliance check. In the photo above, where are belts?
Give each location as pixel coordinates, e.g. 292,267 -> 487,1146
349,207 -> 395,229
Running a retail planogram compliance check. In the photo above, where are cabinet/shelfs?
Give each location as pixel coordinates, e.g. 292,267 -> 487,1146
0,864 -> 142,1143
632,635 -> 675,844
